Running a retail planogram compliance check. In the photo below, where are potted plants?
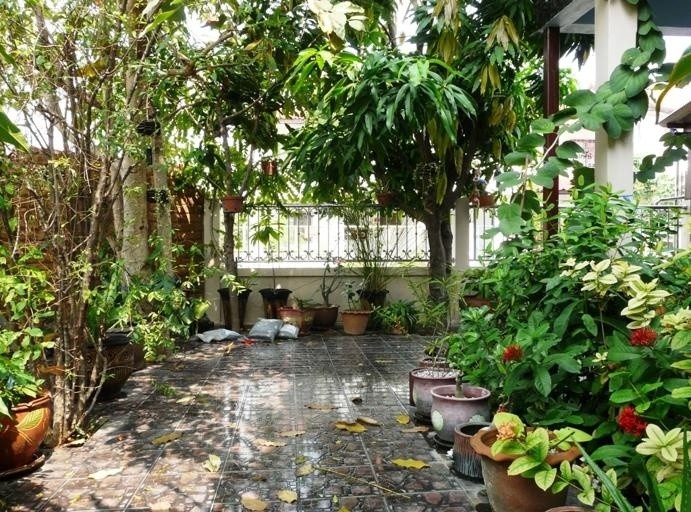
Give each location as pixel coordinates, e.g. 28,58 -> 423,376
411,333 -> 452,408
253,153 -> 285,176
478,313 -> 588,512
0,238 -> 51,482
277,292 -> 314,330
190,153 -> 246,212
545,458 -> 623,512
429,333 -> 492,443
343,208 -> 429,311
217,268 -> 258,332
258,243 -> 293,318
341,271 -> 377,335
403,267 -> 452,367
412,338 -> 464,424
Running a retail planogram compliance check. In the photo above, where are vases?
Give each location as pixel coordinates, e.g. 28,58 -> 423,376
454,423 -> 493,478
